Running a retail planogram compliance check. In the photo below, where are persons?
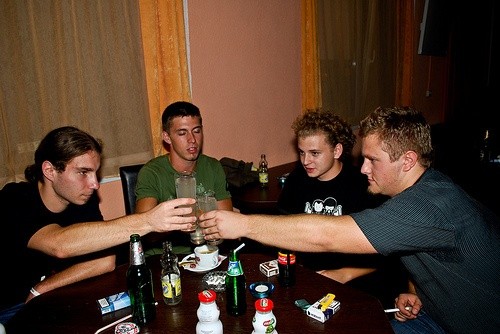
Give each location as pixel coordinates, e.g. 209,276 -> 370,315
134,101 -> 233,257
234,109 -> 384,288
0,126 -> 197,326
198,103 -> 500,334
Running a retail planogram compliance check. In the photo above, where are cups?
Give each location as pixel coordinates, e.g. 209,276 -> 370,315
174,170 -> 198,230
194,244 -> 219,267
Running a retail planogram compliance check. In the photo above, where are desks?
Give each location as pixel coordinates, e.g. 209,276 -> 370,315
232,160 -> 298,215
6,253 -> 396,334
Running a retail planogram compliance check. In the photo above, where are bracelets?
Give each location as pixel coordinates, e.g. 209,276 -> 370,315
30,288 -> 40,296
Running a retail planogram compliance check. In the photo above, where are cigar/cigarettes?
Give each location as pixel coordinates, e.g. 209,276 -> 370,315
383,306 -> 411,313
234,243 -> 244,253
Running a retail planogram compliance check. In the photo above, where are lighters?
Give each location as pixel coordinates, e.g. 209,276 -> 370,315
317,294 -> 335,311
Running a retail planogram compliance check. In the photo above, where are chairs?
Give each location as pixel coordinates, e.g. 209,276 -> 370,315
119,164 -> 144,215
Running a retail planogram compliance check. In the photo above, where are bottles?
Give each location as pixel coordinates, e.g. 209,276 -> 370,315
195,290 -> 223,334
278,248 -> 297,286
251,298 -> 279,334
160,241 -> 183,306
125,234 -> 159,329
258,154 -> 271,184
224,250 -> 247,317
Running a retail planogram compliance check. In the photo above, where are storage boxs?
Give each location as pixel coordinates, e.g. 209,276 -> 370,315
259,259 -> 288,277
306,298 -> 341,322
98,292 -> 131,314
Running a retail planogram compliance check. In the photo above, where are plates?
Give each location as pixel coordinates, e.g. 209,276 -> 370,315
180,253 -> 227,273
